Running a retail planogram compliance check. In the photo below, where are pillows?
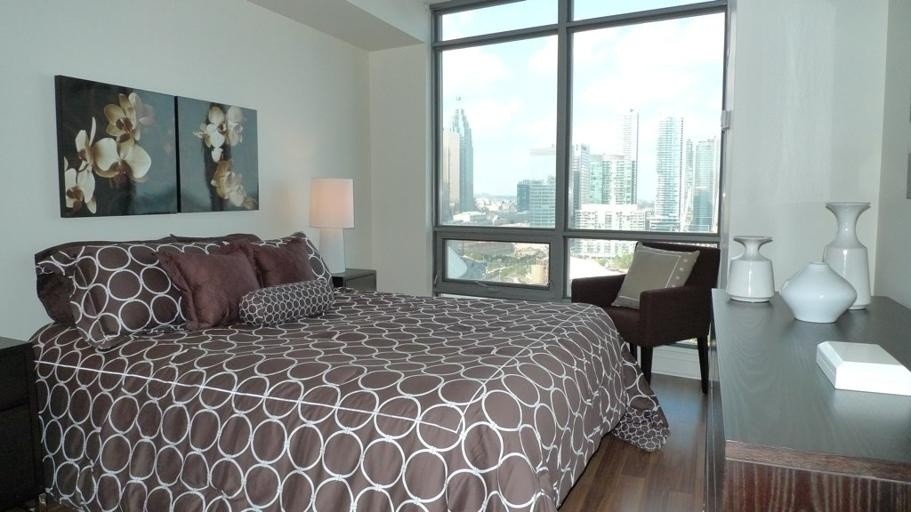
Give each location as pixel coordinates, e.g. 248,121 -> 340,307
251,232 -> 333,292
154,240 -> 264,331
611,240 -> 702,310
238,278 -> 336,327
34,237 -> 185,349
250,235 -> 320,287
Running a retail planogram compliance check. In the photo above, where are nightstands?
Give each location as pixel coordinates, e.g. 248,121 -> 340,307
1,337 -> 41,512
333,268 -> 377,290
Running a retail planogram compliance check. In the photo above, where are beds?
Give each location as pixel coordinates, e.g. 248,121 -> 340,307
26,232 -> 671,511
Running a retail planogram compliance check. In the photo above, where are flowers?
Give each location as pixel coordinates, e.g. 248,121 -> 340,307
65,91 -> 159,214
191,105 -> 258,210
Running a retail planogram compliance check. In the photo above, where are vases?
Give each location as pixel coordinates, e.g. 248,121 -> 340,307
779,260 -> 859,325
825,200 -> 874,311
728,234 -> 775,304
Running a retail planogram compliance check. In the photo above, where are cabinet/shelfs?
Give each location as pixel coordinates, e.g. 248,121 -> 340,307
703,288 -> 911,512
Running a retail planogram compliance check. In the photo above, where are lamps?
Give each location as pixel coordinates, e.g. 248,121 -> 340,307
310,176 -> 355,273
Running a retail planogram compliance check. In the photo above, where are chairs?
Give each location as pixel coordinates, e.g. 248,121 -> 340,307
572,236 -> 722,396
